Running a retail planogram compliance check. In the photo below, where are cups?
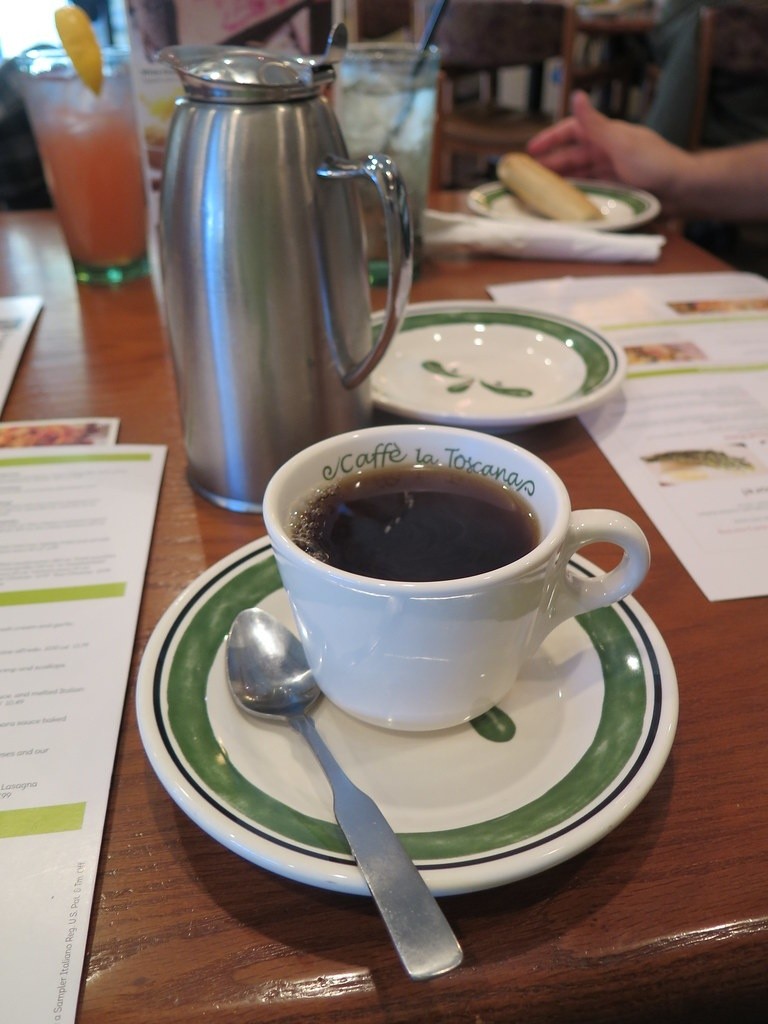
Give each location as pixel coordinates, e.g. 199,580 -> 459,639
331,43 -> 443,287
263,423 -> 651,732
16,46 -> 150,285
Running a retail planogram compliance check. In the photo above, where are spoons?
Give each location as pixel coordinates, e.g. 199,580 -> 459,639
225,608 -> 464,982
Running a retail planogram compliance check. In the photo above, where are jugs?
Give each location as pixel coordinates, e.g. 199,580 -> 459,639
155,22 -> 414,516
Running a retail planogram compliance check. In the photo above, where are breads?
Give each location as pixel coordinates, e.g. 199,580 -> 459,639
496,150 -> 605,224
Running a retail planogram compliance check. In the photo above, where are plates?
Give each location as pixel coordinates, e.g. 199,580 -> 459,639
466,177 -> 660,233
134,532 -> 679,895
370,298 -> 628,437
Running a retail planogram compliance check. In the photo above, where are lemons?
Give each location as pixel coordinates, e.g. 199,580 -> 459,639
54,6 -> 102,96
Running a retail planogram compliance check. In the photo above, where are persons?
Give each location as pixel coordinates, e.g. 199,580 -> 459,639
524,88 -> 768,224
576,0 -> 700,139
